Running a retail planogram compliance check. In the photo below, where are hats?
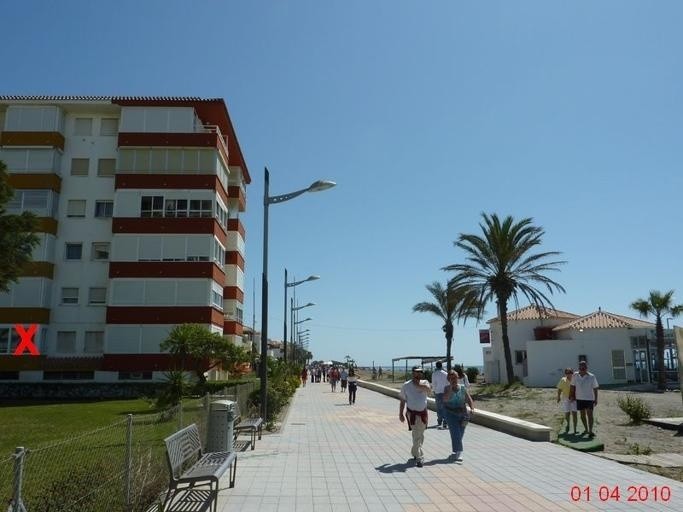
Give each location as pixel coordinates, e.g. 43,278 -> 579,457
412,365 -> 423,372
579,360 -> 588,370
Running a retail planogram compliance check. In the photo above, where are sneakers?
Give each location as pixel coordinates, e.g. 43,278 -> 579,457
414,455 -> 424,467
454,451 -> 464,464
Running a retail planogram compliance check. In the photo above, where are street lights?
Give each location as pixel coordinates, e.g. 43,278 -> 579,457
282,268 -> 321,375
260,165 -> 338,423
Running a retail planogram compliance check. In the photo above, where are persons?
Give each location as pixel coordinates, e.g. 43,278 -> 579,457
301,362 -> 358,405
431,361 -> 450,429
453,364 -> 471,393
399,368 -> 430,467
569,360 -> 599,438
442,369 -> 474,461
555,368 -> 577,433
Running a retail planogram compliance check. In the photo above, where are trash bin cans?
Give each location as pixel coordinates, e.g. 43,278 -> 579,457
207,400 -> 236,452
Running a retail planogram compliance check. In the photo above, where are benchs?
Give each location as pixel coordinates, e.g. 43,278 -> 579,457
163,423 -> 238,512
233,402 -> 263,450
